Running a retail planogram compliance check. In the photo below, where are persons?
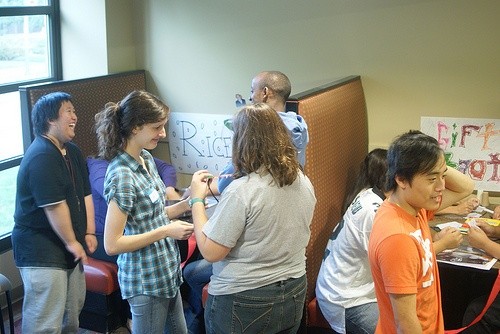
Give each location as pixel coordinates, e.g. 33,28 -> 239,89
182,258 -> 214,334
95,89 -> 209,334
433,197 -> 481,216
84,106 -> 181,278
369,129 -> 475,334
206,70 -> 309,196
466,205 -> 500,334
11,92 -> 98,334
189,103 -> 317,334
315,148 -> 463,334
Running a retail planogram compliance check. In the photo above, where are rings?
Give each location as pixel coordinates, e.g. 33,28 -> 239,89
186,236 -> 188,239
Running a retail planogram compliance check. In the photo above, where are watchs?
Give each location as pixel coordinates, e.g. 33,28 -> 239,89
188,198 -> 205,208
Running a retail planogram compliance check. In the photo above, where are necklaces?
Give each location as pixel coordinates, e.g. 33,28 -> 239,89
391,194 -> 400,207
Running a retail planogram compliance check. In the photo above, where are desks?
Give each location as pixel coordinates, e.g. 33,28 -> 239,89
426,204 -> 500,330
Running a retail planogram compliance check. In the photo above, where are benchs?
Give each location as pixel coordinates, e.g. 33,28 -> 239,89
18,69 -> 146,334
176,74 -> 369,334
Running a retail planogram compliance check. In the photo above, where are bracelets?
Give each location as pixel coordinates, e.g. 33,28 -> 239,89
85,233 -> 96,236
